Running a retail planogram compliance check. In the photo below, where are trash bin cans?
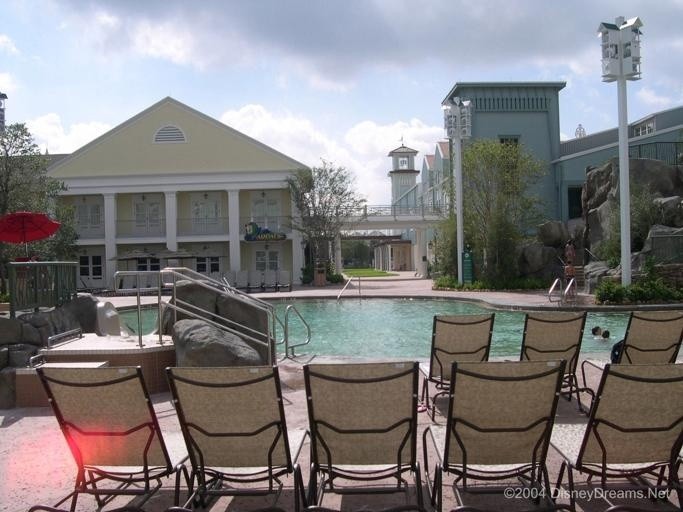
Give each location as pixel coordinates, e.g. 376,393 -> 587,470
314,261 -> 327,287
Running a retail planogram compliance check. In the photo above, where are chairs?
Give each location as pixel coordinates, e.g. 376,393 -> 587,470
418,312 -> 496,421
164,365 -> 312,512
504,312 -> 595,417
422,359 -> 567,512
581,309 -> 683,394
550,363 -> 683,512
302,361 -> 423,512
93,270 -> 293,292
27,366 -> 190,512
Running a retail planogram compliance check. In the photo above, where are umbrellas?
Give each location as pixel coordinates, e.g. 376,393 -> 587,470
1,211 -> 61,259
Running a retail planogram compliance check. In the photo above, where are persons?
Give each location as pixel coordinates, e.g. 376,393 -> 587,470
564,260 -> 575,285
592,326 -> 601,335
564,239 -> 575,261
602,330 -> 609,338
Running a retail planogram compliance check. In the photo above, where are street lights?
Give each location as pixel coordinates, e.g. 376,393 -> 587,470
595,13 -> 645,288
440,95 -> 475,288
0,91 -> 11,298
